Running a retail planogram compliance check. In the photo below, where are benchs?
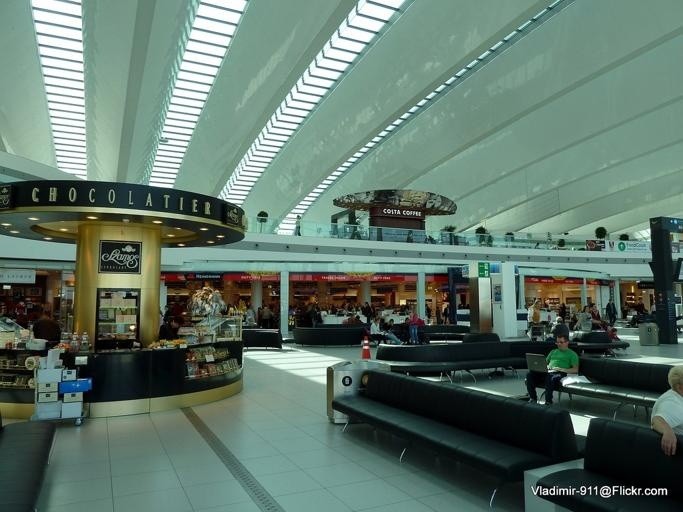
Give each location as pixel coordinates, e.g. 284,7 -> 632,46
242,326 -> 282,349
2,419 -> 58,511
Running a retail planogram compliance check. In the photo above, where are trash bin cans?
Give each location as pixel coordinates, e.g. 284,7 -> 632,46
638,323 -> 659,346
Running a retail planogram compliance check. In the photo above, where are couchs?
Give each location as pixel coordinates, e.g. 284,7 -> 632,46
293,323 -> 682,510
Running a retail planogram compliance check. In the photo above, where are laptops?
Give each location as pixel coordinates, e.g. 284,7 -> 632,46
525,352 -> 558,374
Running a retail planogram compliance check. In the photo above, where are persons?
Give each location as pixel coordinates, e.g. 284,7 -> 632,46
527,298 -> 656,340
258,300 -> 449,344
294,215 -> 301,236
525,333 -> 579,405
649,365 -> 683,455
158,317 -> 184,338
163,304 -> 169,322
32,309 -> 60,341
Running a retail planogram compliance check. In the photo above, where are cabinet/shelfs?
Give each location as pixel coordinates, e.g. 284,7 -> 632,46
185,342 -> 243,380
96,290 -> 139,350
0,353 -> 47,403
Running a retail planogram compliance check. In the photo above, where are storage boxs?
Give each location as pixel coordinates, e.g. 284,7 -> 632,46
35,350 -> 94,422
